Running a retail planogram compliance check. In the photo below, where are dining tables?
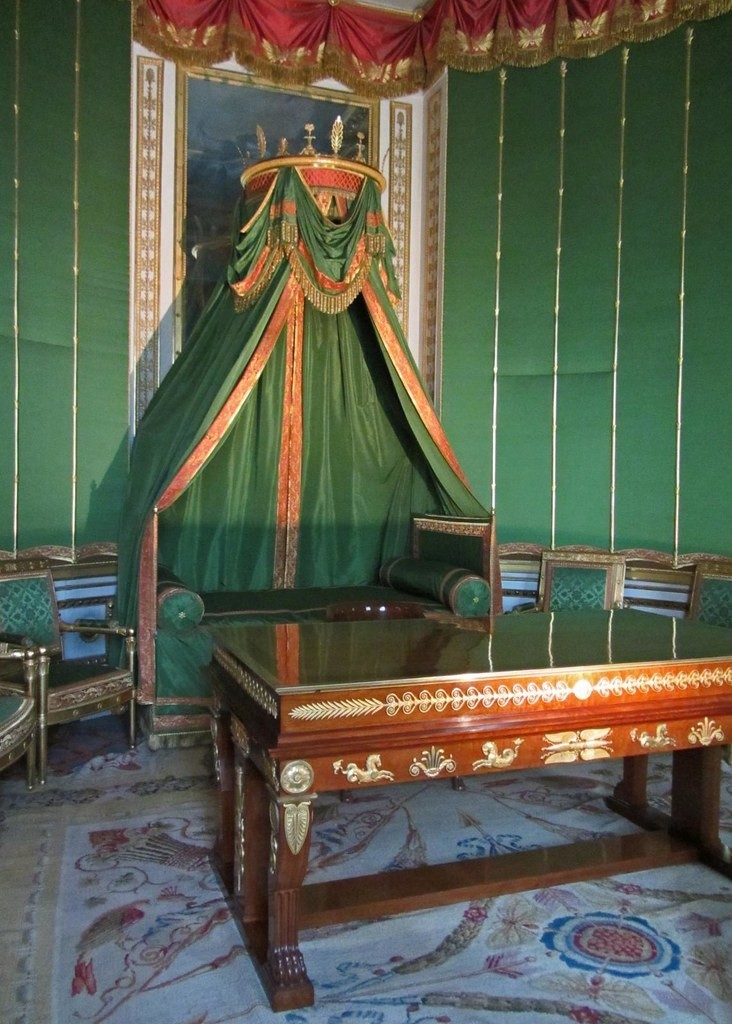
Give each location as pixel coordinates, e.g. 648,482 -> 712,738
198,605 -> 732,1014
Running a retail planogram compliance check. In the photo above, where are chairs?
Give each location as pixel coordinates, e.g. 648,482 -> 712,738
683,557 -> 732,628
0,557 -> 139,786
505,546 -> 628,614
1,631 -> 43,791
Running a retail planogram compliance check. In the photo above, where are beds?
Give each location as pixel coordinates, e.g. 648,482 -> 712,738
128,511 -> 504,753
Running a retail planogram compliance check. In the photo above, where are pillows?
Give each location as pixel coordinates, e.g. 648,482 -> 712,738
156,562 -> 205,635
379,554 -> 492,619
